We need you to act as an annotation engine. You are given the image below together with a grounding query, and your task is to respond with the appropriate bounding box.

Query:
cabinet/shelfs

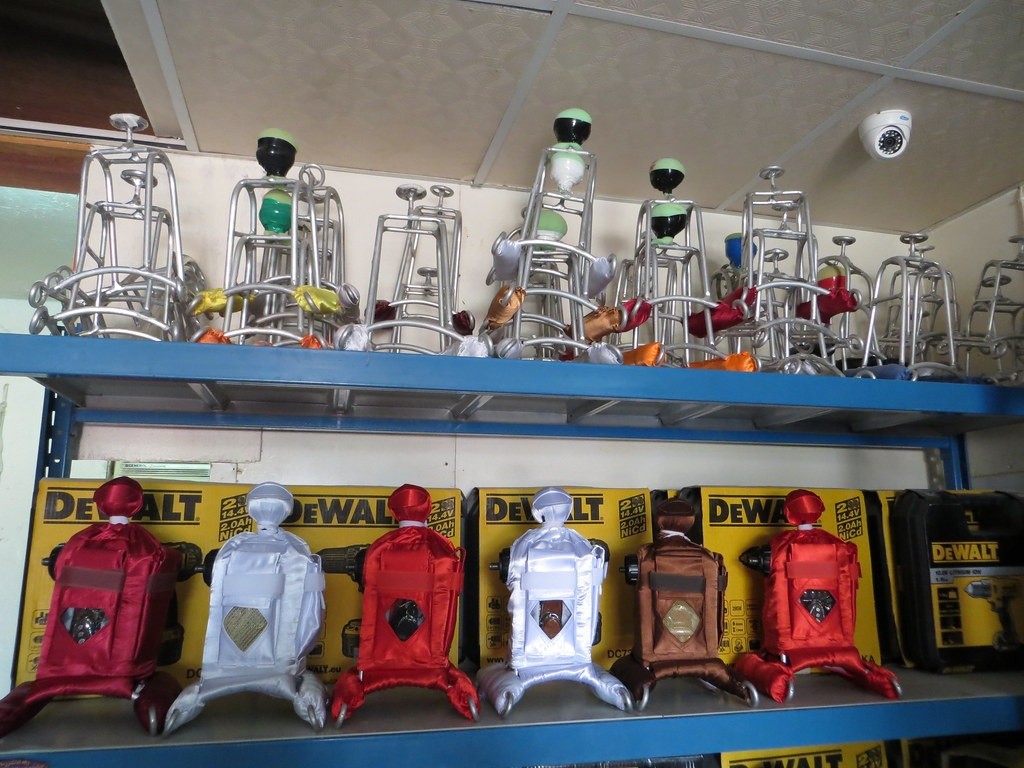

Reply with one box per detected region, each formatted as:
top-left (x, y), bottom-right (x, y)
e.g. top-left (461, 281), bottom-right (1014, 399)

top-left (0, 332), bottom-right (1024, 768)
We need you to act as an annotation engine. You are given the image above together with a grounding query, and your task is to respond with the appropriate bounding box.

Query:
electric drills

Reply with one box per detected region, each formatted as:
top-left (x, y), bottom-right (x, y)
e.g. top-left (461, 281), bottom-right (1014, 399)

top-left (41, 545), bottom-right (62, 581)
top-left (344, 549), bottom-right (364, 591)
top-left (489, 546), bottom-right (511, 583)
top-left (619, 554), bottom-right (639, 584)
top-left (737, 542), bottom-right (772, 575)
top-left (194, 547), bottom-right (220, 588)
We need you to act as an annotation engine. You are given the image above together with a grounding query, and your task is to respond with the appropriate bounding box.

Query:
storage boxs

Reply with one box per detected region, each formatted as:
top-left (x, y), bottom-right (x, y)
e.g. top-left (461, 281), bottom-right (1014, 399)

top-left (15, 477), bottom-right (258, 701)
top-left (466, 486), bottom-right (653, 671)
top-left (279, 486), bottom-right (461, 685)
top-left (679, 485), bottom-right (882, 674)
top-left (862, 490), bottom-right (1001, 667)
top-left (703, 740), bottom-right (888, 768)
top-left (650, 489), bottom-right (682, 542)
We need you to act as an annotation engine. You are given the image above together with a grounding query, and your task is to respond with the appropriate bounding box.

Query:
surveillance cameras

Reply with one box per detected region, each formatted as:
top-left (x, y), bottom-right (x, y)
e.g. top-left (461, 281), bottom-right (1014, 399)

top-left (858, 109), bottom-right (912, 160)
